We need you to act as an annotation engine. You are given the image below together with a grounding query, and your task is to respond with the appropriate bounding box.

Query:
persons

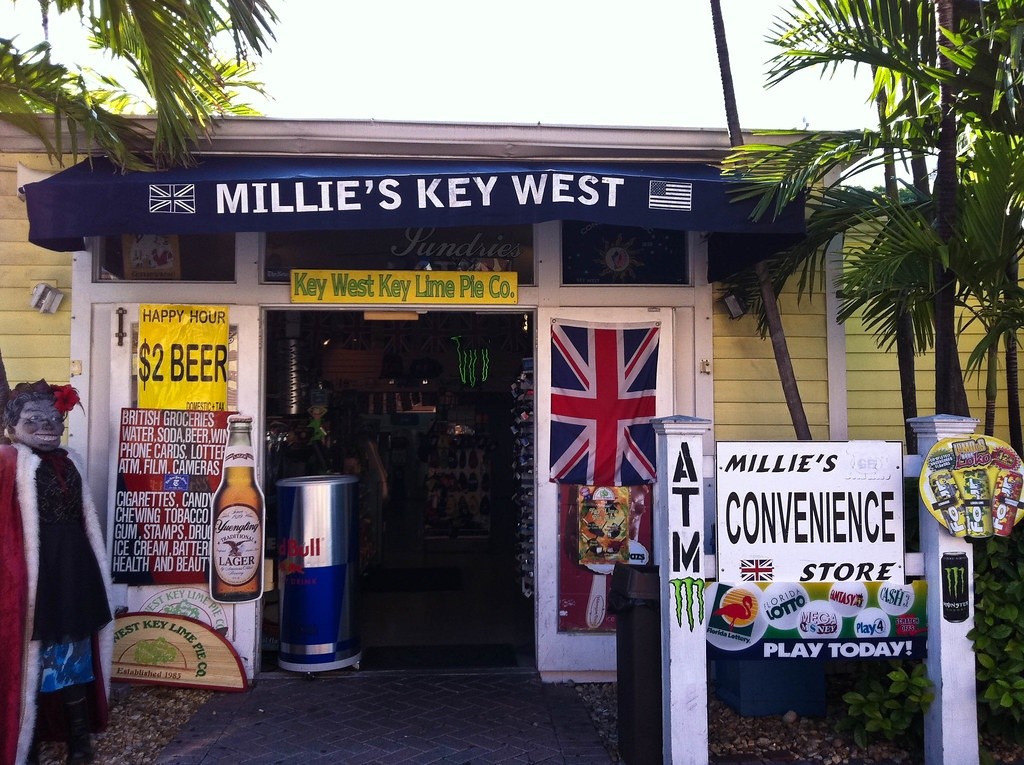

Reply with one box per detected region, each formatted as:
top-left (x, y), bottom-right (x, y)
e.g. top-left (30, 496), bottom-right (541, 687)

top-left (3, 383), bottom-right (65, 451)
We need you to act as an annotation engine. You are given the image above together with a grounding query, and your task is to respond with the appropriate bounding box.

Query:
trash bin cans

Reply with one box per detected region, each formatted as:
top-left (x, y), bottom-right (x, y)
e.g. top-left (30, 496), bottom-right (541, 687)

top-left (606, 558), bottom-right (663, 765)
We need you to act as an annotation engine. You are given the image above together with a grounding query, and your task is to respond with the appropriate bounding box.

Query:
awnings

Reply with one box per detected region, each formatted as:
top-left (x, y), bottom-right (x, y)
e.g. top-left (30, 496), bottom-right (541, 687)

top-left (18, 153), bottom-right (809, 254)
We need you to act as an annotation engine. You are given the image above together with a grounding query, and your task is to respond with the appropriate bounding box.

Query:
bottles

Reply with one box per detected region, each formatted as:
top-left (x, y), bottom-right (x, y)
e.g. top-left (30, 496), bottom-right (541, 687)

top-left (206, 416), bottom-right (266, 604)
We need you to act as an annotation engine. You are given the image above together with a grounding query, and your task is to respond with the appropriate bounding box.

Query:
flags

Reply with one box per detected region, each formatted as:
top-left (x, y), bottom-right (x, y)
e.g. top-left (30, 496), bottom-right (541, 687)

top-left (549, 316), bottom-right (660, 486)
